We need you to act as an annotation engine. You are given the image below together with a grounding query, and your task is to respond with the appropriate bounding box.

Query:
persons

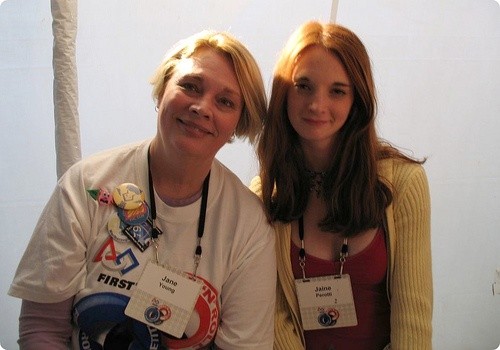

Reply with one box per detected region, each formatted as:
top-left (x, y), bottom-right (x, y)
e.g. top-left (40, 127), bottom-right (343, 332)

top-left (247, 19), bottom-right (434, 350)
top-left (6, 29), bottom-right (277, 350)
